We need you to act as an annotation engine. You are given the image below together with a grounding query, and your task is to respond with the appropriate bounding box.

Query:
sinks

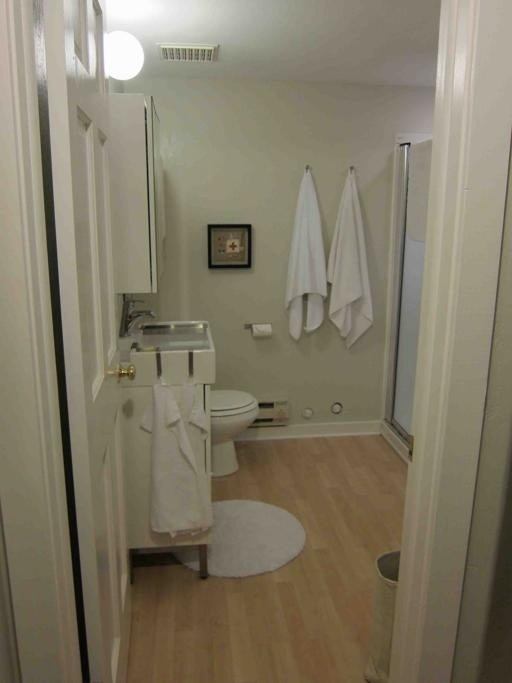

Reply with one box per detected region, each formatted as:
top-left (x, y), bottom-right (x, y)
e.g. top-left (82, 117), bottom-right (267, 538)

top-left (141, 323), bottom-right (206, 335)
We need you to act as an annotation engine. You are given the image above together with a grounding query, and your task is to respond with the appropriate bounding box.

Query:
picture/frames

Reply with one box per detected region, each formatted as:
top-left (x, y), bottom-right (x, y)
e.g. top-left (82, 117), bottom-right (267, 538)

top-left (208, 222), bottom-right (252, 271)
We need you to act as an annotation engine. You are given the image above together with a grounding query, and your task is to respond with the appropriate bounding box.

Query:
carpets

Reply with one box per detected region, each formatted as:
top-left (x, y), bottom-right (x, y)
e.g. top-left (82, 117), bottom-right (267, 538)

top-left (167, 497), bottom-right (307, 576)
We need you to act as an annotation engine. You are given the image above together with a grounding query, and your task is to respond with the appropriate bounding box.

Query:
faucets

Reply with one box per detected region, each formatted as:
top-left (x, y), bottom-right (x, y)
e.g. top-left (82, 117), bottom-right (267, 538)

top-left (121, 300), bottom-right (156, 337)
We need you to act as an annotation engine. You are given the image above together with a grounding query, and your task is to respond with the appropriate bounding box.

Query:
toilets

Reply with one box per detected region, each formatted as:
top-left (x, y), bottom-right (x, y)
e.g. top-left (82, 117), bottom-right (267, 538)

top-left (209, 389), bottom-right (259, 478)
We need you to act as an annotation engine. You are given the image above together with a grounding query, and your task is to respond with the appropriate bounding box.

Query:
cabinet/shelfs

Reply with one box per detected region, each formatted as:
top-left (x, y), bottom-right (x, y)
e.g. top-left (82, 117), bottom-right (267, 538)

top-left (111, 91), bottom-right (163, 293)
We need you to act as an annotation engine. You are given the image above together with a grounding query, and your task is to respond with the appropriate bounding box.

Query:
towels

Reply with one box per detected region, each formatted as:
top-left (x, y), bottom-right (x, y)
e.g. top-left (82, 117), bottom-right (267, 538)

top-left (282, 161), bottom-right (372, 351)
top-left (138, 370), bottom-right (211, 537)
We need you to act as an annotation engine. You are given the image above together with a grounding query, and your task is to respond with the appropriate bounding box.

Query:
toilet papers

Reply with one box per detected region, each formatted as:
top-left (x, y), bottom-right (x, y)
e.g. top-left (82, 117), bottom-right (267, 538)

top-left (251, 323), bottom-right (272, 337)
